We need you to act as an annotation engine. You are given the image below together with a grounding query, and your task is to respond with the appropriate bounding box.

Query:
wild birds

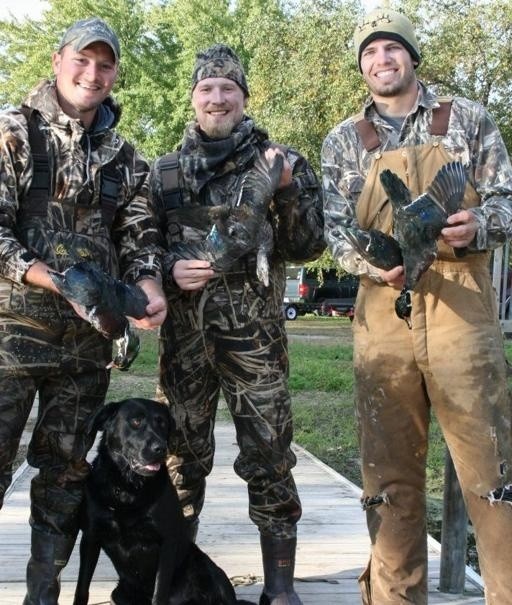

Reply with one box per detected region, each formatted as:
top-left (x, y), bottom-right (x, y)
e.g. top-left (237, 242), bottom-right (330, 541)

top-left (172, 150), bottom-right (285, 290)
top-left (334, 162), bottom-right (468, 331)
top-left (44, 230), bottom-right (150, 371)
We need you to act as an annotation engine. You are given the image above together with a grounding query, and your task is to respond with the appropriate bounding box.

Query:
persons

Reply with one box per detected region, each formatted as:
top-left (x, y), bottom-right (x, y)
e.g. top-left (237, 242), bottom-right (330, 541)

top-left (314, 7), bottom-right (511, 604)
top-left (0, 13), bottom-right (173, 605)
top-left (136, 40), bottom-right (328, 605)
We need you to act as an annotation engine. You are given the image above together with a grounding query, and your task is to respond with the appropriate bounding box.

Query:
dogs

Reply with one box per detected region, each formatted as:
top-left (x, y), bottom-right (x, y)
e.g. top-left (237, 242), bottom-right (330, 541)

top-left (72, 396), bottom-right (271, 604)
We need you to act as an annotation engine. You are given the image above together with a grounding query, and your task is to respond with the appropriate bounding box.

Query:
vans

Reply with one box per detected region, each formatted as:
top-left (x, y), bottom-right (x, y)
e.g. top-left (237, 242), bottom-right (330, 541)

top-left (282, 266), bottom-right (359, 307)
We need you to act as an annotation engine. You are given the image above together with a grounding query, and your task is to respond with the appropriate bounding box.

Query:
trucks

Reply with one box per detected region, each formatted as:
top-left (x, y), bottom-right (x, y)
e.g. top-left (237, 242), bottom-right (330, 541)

top-left (320, 298), bottom-right (355, 322)
top-left (280, 295), bottom-right (308, 321)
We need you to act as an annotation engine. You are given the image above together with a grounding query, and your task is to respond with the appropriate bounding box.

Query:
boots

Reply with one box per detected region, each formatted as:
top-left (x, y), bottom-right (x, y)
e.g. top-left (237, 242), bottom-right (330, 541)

top-left (258, 536), bottom-right (301, 605)
top-left (22, 525), bottom-right (77, 605)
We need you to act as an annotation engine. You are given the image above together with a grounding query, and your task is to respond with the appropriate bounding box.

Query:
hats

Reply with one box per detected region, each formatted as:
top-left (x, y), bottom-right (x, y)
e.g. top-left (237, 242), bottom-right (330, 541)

top-left (58, 18), bottom-right (121, 63)
top-left (354, 8), bottom-right (420, 74)
top-left (190, 43), bottom-right (250, 96)
